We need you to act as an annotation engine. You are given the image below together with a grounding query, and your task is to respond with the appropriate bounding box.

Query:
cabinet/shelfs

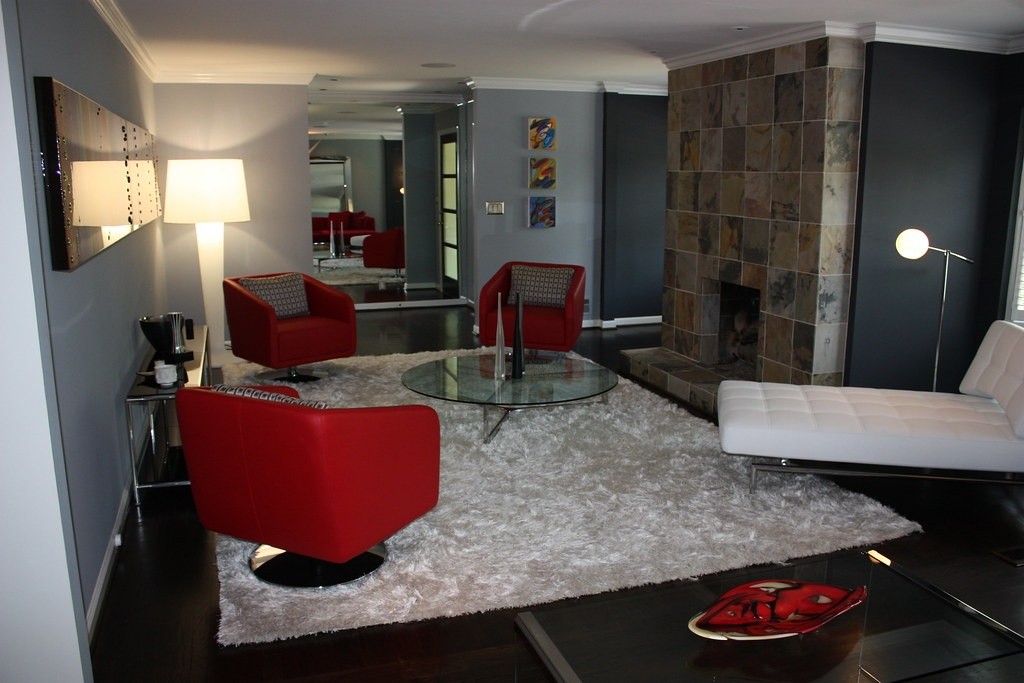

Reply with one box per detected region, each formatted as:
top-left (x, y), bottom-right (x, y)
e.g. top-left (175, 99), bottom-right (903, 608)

top-left (512, 546), bottom-right (1023, 683)
top-left (125, 324), bottom-right (210, 525)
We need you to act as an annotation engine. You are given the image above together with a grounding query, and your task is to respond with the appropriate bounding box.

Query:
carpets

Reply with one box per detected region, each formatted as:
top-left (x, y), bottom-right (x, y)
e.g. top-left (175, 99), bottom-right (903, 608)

top-left (217, 345), bottom-right (922, 643)
top-left (313, 249), bottom-right (405, 285)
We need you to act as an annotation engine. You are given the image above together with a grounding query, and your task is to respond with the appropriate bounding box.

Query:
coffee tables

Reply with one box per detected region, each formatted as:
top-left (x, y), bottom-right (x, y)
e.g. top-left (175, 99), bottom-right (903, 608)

top-left (312, 251), bottom-right (363, 271)
top-left (401, 353), bottom-right (618, 443)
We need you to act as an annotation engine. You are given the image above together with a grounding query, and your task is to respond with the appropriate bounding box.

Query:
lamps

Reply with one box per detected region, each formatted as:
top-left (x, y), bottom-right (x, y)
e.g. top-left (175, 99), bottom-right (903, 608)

top-left (69, 158), bottom-right (162, 248)
top-left (164, 157), bottom-right (252, 371)
top-left (895, 228), bottom-right (973, 392)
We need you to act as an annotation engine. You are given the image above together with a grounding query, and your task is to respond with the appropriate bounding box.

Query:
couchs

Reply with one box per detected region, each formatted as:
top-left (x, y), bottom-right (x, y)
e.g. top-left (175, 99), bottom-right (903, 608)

top-left (176, 381), bottom-right (440, 587)
top-left (312, 214), bottom-right (375, 245)
top-left (224, 270), bottom-right (357, 382)
top-left (715, 318), bottom-right (1023, 493)
top-left (363, 228), bottom-right (406, 278)
top-left (478, 260), bottom-right (588, 365)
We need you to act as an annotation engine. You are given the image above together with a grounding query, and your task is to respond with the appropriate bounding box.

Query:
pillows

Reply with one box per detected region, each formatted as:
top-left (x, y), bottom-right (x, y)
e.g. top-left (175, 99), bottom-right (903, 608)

top-left (239, 272), bottom-right (309, 318)
top-left (210, 383), bottom-right (331, 411)
top-left (327, 210), bottom-right (352, 230)
top-left (349, 211), bottom-right (365, 229)
top-left (509, 265), bottom-right (576, 308)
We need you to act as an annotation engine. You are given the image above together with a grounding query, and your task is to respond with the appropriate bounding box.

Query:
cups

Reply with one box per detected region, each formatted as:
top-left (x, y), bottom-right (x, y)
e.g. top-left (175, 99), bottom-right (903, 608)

top-left (154, 365), bottom-right (177, 386)
